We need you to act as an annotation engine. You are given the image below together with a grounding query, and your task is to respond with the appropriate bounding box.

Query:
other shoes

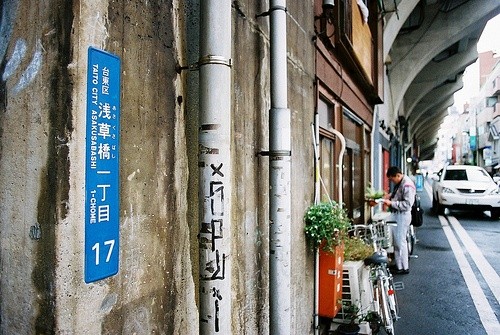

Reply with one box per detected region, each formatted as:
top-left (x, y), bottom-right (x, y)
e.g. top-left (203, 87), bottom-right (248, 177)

top-left (393, 269), bottom-right (408, 275)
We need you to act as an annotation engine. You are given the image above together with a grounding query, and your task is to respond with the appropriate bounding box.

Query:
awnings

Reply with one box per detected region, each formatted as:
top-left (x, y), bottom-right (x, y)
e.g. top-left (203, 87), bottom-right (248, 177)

top-left (494, 164), bottom-right (500, 169)
top-left (490, 162), bottom-right (498, 165)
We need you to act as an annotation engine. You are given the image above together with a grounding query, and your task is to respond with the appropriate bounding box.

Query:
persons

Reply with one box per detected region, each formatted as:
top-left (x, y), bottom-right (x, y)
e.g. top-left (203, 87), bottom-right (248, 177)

top-left (380, 166), bottom-right (416, 274)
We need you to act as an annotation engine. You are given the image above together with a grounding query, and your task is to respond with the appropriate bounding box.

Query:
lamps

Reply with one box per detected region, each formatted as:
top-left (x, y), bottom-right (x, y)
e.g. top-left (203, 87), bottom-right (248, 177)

top-left (314, 0), bottom-right (335, 24)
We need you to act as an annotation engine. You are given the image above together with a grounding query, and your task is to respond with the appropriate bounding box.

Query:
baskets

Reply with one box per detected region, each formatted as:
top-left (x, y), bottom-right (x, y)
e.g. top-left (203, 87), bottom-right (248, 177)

top-left (356, 224), bottom-right (389, 249)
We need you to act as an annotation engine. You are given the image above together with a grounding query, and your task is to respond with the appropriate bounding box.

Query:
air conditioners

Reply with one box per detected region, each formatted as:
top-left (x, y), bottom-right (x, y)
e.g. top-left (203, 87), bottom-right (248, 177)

top-left (353, 224), bottom-right (377, 255)
top-left (332, 260), bottom-right (374, 324)
top-left (373, 219), bottom-right (393, 248)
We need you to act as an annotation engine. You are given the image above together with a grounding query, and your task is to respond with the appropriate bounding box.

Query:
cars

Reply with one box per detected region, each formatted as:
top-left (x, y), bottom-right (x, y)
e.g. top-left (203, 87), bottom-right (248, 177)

top-left (428, 164), bottom-right (500, 221)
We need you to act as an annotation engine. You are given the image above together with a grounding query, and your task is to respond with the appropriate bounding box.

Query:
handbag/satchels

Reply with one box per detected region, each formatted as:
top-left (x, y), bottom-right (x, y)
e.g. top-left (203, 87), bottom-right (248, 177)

top-left (412, 206), bottom-right (424, 226)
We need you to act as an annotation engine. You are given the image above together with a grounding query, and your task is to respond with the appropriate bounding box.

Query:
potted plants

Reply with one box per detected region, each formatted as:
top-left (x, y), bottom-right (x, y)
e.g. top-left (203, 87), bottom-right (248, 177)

top-left (365, 183), bottom-right (385, 206)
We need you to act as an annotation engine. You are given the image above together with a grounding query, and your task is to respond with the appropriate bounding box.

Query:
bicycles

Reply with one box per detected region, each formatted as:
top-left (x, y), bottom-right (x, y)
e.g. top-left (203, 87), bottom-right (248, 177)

top-left (347, 219), bottom-right (404, 335)
top-left (406, 223), bottom-right (420, 260)
